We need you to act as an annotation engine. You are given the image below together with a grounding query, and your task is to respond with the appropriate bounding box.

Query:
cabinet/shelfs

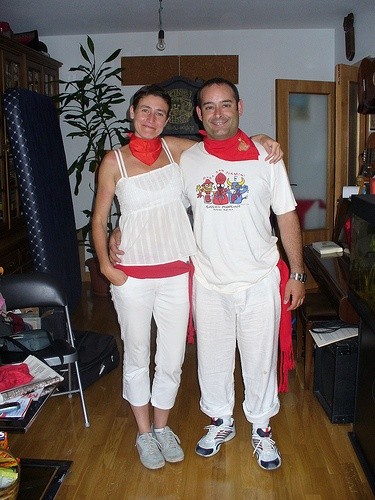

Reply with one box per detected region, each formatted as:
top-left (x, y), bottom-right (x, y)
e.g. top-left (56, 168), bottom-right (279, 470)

top-left (0, 34), bottom-right (63, 278)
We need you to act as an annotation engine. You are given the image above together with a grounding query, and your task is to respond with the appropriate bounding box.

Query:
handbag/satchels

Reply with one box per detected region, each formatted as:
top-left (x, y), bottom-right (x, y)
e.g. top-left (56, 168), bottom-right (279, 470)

top-left (57, 330), bottom-right (120, 395)
top-left (0, 322), bottom-right (64, 372)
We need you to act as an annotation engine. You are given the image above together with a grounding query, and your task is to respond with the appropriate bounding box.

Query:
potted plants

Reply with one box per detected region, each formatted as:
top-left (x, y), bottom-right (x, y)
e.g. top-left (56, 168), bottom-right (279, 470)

top-left (46, 35), bottom-right (135, 297)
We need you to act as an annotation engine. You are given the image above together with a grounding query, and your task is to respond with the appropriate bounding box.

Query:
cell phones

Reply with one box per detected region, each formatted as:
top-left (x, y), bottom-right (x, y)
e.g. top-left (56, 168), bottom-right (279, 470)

top-left (0, 402), bottom-right (20, 414)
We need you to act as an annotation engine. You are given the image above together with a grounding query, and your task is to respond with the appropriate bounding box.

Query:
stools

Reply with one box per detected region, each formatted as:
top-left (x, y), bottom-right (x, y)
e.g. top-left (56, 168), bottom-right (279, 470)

top-left (296, 292), bottom-right (338, 390)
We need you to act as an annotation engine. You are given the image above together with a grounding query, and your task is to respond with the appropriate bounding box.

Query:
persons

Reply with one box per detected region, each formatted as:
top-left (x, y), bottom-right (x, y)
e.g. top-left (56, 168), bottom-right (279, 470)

top-left (92, 86), bottom-right (286, 471)
top-left (107, 79), bottom-right (306, 473)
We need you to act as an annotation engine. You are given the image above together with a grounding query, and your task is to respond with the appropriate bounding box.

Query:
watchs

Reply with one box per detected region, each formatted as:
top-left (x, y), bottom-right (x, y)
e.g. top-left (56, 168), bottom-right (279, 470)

top-left (289, 273), bottom-right (308, 283)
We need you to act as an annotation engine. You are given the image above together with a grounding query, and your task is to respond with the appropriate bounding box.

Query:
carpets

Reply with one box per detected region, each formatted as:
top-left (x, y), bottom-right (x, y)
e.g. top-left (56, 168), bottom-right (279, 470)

top-left (16, 458), bottom-right (73, 500)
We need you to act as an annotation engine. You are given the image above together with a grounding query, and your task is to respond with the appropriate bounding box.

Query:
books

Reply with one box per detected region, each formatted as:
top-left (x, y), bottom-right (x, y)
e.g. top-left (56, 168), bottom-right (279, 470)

top-left (311, 240), bottom-right (343, 254)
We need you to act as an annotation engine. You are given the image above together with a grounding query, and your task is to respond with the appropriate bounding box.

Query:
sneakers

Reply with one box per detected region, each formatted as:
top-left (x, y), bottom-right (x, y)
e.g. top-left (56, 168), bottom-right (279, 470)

top-left (251, 424), bottom-right (282, 470)
top-left (151, 423), bottom-right (185, 462)
top-left (194, 417), bottom-right (236, 458)
top-left (133, 430), bottom-right (170, 470)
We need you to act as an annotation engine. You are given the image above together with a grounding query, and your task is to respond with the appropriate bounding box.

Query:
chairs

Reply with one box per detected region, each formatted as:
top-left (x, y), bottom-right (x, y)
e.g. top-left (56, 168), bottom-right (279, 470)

top-left (0, 272), bottom-right (91, 429)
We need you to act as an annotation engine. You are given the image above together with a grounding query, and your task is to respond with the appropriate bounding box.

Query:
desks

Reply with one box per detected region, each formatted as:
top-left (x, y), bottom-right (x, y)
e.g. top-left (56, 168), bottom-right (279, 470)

top-left (303, 243), bottom-right (360, 325)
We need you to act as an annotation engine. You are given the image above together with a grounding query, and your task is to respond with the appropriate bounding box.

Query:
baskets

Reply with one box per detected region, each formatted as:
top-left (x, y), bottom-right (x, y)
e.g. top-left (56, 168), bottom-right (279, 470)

top-left (0, 448), bottom-right (21, 500)
